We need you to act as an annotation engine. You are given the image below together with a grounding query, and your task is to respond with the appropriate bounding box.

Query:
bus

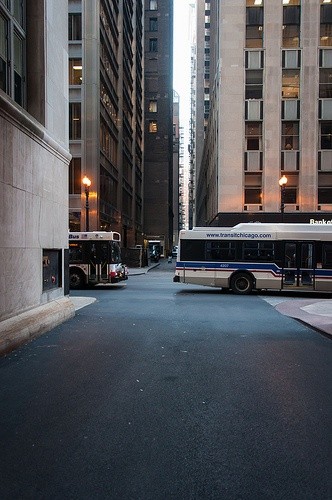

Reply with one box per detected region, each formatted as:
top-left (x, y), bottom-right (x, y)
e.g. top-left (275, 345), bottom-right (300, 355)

top-left (68, 231), bottom-right (129, 290)
top-left (172, 222), bottom-right (332, 296)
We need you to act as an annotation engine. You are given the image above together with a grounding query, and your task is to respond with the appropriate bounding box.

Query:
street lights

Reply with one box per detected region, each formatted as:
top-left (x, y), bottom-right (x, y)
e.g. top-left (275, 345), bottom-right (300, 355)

top-left (81, 176), bottom-right (92, 231)
top-left (278, 175), bottom-right (288, 222)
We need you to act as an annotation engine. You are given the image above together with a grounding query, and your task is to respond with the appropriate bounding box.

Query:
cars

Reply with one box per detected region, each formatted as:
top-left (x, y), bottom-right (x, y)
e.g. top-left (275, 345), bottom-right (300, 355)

top-left (172, 245), bottom-right (178, 258)
top-left (150, 250), bottom-right (160, 263)
